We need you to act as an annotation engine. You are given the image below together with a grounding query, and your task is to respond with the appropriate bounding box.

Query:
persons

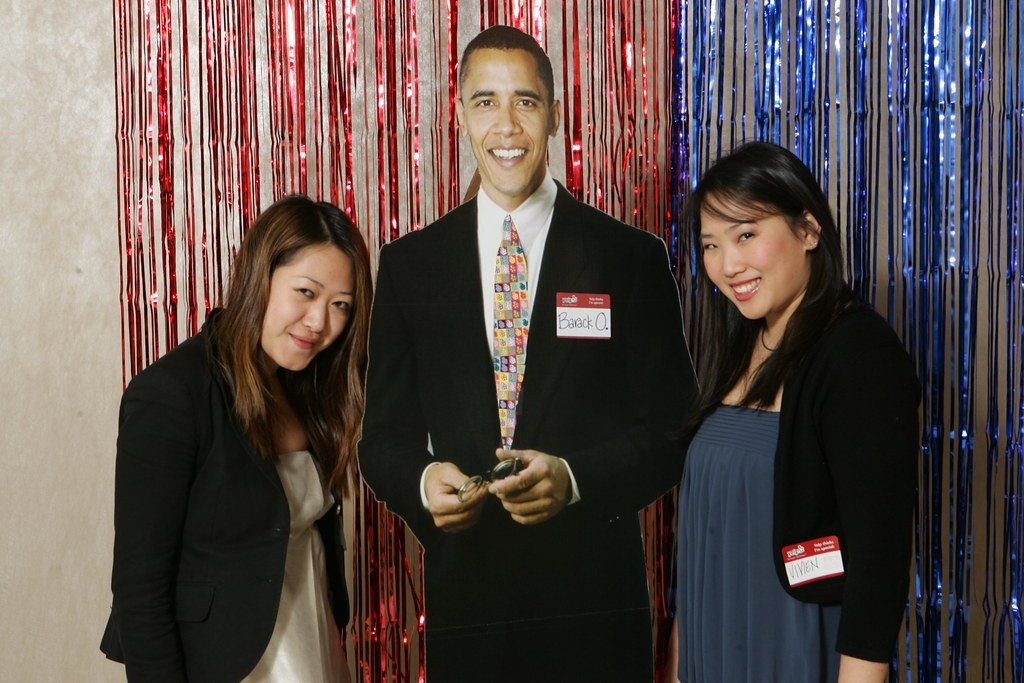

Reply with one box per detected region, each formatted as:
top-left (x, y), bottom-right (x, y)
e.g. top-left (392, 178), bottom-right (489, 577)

top-left (97, 193), bottom-right (372, 683)
top-left (360, 25), bottom-right (710, 683)
top-left (662, 139), bottom-right (924, 683)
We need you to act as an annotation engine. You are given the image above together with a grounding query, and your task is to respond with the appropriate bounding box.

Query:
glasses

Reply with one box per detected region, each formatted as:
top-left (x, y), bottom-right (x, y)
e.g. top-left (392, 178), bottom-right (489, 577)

top-left (457, 458), bottom-right (520, 502)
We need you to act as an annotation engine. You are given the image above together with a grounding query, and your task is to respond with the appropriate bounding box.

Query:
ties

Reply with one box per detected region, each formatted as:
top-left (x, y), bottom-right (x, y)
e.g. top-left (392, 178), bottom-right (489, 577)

top-left (493, 214), bottom-right (531, 451)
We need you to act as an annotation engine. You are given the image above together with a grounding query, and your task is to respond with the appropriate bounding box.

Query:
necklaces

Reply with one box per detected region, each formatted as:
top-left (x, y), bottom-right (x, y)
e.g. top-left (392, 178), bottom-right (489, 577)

top-left (761, 322), bottom-right (777, 352)
